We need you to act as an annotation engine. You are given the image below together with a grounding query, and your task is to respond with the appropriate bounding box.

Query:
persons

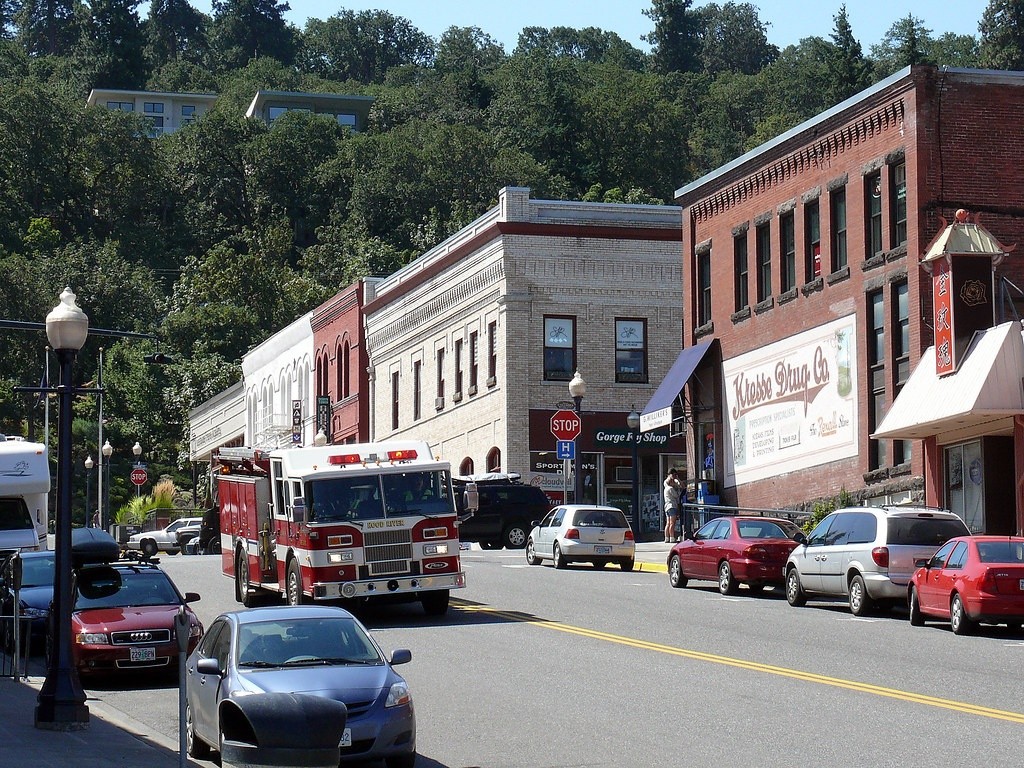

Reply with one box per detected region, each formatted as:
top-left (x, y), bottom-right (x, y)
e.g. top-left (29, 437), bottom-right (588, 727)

top-left (664, 467), bottom-right (684, 543)
top-left (93, 510), bottom-right (99, 528)
top-left (401, 475), bottom-right (433, 501)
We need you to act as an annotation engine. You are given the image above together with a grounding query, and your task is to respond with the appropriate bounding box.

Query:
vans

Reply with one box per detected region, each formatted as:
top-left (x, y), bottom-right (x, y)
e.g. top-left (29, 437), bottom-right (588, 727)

top-left (0, 431), bottom-right (51, 565)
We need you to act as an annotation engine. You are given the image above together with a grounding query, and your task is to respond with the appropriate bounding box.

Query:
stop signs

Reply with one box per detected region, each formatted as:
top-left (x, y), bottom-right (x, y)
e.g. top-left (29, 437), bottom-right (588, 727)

top-left (131, 468), bottom-right (148, 485)
top-left (550, 410), bottom-right (581, 442)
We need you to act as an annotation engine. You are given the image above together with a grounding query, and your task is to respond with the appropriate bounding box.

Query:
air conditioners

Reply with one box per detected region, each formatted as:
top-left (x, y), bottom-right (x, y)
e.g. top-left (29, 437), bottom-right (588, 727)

top-left (435, 396), bottom-right (444, 409)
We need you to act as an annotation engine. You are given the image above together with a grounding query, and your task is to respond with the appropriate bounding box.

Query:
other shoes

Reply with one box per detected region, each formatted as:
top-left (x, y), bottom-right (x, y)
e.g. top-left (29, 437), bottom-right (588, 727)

top-left (670, 540), bottom-right (680, 543)
top-left (665, 540), bottom-right (670, 543)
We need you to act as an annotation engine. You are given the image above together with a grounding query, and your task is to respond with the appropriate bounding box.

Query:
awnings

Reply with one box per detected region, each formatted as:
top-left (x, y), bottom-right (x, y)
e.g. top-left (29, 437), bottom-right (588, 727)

top-left (640, 337), bottom-right (714, 432)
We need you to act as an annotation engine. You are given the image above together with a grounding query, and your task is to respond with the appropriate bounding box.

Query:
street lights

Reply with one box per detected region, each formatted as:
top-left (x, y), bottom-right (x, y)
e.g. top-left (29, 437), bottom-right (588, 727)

top-left (33, 282), bottom-right (92, 731)
top-left (101, 438), bottom-right (113, 533)
top-left (568, 371), bottom-right (588, 505)
top-left (84, 454), bottom-right (94, 528)
top-left (132, 441), bottom-right (143, 498)
top-left (626, 403), bottom-right (645, 543)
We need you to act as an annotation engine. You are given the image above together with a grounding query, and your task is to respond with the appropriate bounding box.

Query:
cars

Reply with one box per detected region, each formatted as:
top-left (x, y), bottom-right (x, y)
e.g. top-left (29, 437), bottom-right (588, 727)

top-left (44, 527), bottom-right (204, 683)
top-left (905, 536), bottom-right (1024, 636)
top-left (0, 550), bottom-right (57, 657)
top-left (525, 504), bottom-right (636, 573)
top-left (184, 604), bottom-right (416, 768)
top-left (666, 515), bottom-right (808, 597)
top-left (127, 517), bottom-right (203, 557)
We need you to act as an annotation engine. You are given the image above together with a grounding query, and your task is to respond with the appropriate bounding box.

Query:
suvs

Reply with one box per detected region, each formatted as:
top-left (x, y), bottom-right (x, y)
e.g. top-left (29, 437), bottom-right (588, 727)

top-left (785, 504), bottom-right (973, 618)
top-left (441, 479), bottom-right (553, 550)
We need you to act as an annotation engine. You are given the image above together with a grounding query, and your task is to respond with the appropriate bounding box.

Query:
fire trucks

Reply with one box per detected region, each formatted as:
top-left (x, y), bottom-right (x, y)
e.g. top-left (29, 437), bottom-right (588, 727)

top-left (212, 437), bottom-right (467, 618)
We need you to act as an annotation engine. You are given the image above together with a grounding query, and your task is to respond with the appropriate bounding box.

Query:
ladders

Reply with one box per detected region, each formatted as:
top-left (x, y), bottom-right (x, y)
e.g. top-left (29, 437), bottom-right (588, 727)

top-left (219, 445), bottom-right (279, 458)
